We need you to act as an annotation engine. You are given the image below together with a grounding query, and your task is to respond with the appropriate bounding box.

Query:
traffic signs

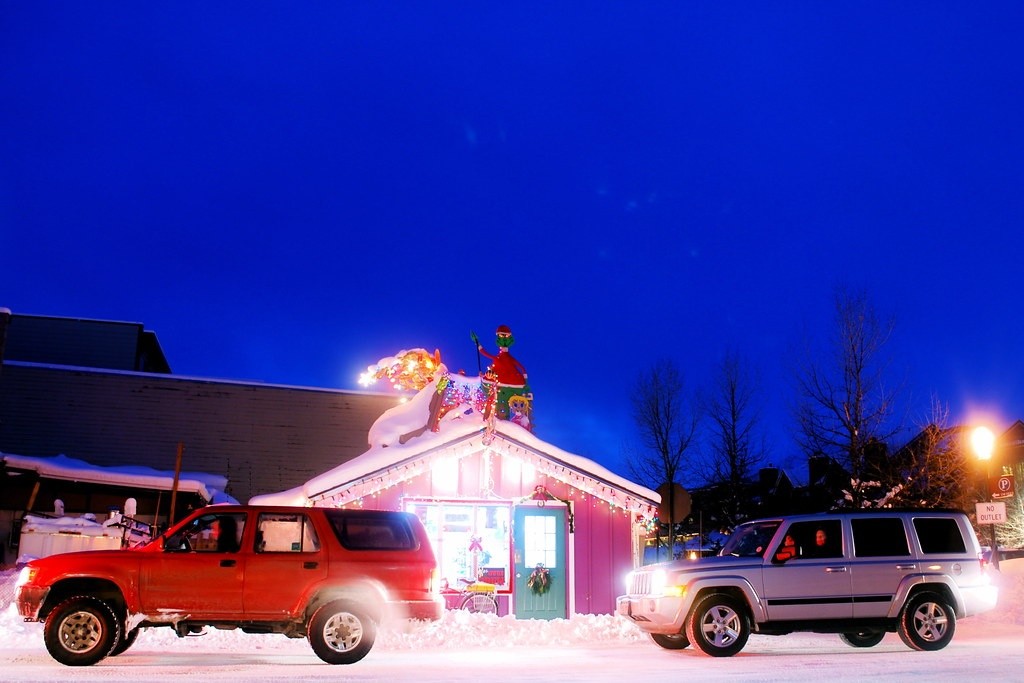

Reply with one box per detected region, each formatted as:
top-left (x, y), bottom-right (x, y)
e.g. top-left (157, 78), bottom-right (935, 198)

top-left (992, 474), bottom-right (1015, 499)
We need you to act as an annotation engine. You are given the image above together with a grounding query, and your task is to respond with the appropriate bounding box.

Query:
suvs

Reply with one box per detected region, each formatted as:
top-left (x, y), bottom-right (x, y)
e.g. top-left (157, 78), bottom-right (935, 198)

top-left (17, 504), bottom-right (445, 668)
top-left (616, 508), bottom-right (1005, 659)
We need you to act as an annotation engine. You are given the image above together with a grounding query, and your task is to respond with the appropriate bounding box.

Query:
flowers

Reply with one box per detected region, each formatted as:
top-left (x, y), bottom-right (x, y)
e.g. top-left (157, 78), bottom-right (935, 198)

top-left (526, 564), bottom-right (555, 596)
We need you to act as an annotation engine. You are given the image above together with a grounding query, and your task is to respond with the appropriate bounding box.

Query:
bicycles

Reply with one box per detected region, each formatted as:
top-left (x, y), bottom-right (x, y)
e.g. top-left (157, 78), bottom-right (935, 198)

top-left (441, 578), bottom-right (499, 616)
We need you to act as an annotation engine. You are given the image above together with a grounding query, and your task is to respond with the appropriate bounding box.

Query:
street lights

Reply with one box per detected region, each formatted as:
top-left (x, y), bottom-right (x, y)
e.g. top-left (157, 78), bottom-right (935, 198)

top-left (971, 426), bottom-right (1000, 571)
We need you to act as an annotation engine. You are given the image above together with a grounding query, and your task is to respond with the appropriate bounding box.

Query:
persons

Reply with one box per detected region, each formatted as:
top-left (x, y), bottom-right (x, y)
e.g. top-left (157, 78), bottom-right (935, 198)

top-left (815, 529), bottom-right (826, 546)
top-left (508, 393), bottom-right (531, 432)
top-left (210, 517), bottom-right (240, 553)
